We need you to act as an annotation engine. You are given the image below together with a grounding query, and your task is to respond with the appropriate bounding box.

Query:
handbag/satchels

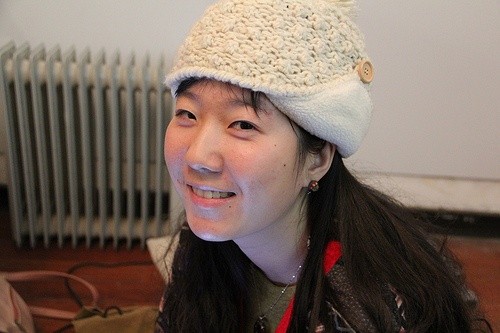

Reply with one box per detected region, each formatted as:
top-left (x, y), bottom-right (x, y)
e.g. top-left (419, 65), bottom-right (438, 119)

top-left (0, 273), bottom-right (36, 333)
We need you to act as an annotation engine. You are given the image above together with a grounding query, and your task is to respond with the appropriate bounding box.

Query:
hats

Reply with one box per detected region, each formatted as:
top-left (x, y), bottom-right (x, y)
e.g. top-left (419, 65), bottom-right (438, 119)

top-left (163, 1), bottom-right (374, 158)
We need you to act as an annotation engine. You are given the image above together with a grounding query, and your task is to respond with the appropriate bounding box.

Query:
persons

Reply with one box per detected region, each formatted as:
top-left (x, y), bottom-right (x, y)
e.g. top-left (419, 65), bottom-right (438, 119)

top-left (158, 1), bottom-right (494, 333)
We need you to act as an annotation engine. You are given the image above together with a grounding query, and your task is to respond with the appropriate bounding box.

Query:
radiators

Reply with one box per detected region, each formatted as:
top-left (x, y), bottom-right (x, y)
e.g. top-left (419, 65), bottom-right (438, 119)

top-left (0, 39), bottom-right (187, 252)
